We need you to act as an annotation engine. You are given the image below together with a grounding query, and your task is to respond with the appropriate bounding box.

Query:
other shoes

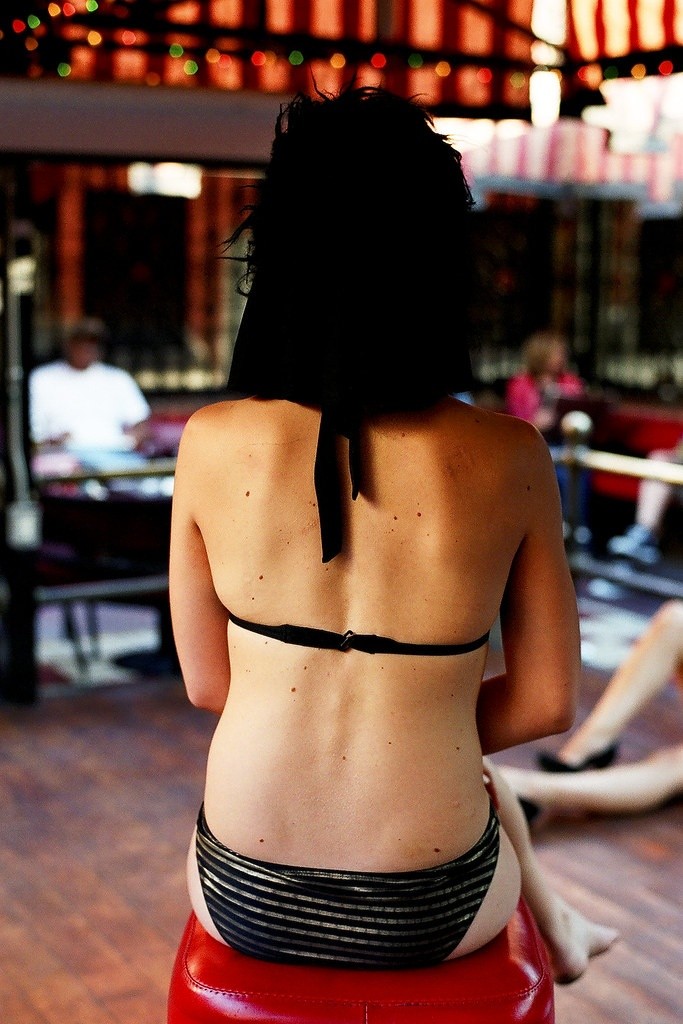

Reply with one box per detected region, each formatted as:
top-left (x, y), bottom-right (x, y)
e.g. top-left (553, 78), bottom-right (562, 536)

top-left (539, 745), bottom-right (613, 773)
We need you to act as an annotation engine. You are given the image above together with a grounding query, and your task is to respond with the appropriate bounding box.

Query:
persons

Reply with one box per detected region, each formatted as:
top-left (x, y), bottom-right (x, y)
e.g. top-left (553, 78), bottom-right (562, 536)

top-left (585, 442), bottom-right (683, 601)
top-left (28, 320), bottom-right (154, 677)
top-left (495, 596), bottom-right (683, 827)
top-left (510, 329), bottom-right (591, 540)
top-left (168, 86), bottom-right (622, 989)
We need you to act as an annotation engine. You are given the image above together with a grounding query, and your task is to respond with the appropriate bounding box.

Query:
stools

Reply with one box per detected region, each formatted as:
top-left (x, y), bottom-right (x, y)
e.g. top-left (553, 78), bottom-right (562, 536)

top-left (166, 895), bottom-right (557, 1023)
